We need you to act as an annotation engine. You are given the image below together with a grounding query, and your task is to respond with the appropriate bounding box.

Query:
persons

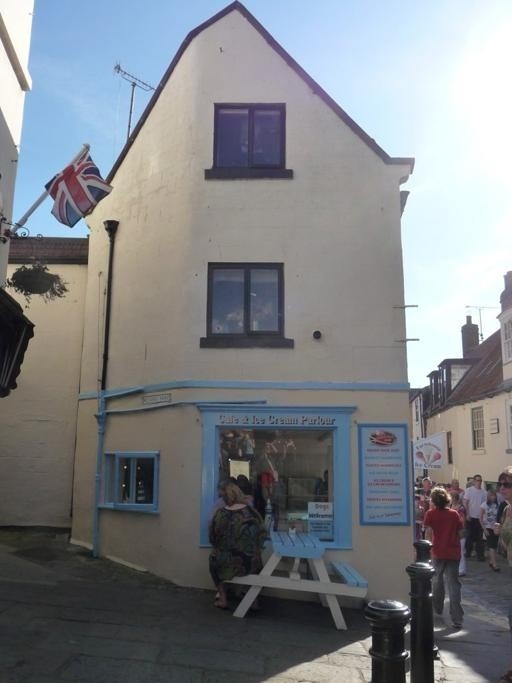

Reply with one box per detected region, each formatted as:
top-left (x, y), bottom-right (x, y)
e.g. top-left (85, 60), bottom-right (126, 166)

top-left (414, 465), bottom-right (512, 683)
top-left (315, 470), bottom-right (328, 502)
top-left (208, 475), bottom-right (267, 610)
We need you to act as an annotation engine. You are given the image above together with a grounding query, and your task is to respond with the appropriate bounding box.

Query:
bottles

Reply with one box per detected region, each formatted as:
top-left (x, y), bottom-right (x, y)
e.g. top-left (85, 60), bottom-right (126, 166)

top-left (264, 498), bottom-right (274, 538)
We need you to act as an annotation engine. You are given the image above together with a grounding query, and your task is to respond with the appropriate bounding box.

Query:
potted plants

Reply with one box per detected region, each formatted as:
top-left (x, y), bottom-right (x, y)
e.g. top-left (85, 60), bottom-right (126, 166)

top-left (3, 263), bottom-right (70, 309)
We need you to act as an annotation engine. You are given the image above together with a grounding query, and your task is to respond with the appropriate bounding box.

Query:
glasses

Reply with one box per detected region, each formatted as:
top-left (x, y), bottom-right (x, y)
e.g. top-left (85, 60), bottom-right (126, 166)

top-left (496, 481), bottom-right (512, 488)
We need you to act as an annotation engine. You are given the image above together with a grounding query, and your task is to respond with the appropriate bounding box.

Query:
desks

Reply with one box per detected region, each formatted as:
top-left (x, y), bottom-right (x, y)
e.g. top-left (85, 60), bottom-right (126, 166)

top-left (233, 531), bottom-right (348, 630)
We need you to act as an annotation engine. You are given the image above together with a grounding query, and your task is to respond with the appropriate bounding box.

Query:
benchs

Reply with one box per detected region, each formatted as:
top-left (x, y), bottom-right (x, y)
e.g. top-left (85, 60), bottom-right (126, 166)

top-left (327, 561), bottom-right (368, 599)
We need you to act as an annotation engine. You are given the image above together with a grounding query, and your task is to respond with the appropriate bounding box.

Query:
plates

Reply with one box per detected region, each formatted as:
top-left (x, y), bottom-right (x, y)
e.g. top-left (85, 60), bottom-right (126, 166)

top-left (368, 431), bottom-right (397, 447)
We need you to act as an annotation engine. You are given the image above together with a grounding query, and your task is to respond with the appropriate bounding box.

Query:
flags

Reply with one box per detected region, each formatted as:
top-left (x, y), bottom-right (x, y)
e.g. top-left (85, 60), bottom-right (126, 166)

top-left (44, 148), bottom-right (114, 228)
